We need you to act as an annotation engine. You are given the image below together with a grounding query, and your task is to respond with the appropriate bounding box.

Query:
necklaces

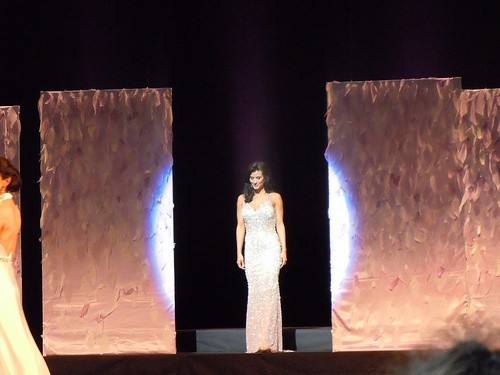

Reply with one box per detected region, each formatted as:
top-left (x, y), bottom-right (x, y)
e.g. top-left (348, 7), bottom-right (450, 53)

top-left (0, 193), bottom-right (13, 201)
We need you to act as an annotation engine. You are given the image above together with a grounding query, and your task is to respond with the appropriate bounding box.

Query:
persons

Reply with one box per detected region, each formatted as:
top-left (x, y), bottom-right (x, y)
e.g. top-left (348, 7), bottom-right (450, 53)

top-left (236, 162), bottom-right (286, 353)
top-left (0, 157), bottom-right (51, 375)
